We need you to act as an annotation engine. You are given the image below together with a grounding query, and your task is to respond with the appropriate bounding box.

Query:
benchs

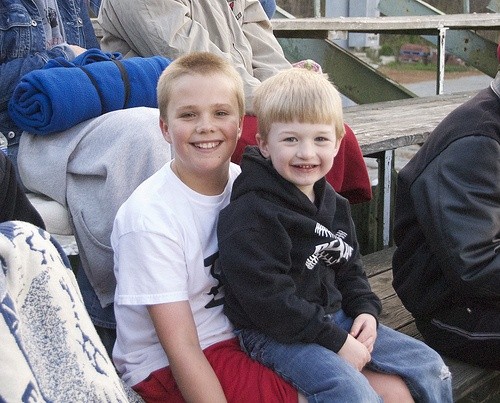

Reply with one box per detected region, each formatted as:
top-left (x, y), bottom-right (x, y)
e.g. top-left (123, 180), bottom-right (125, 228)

top-left (50, 13), bottom-right (500, 403)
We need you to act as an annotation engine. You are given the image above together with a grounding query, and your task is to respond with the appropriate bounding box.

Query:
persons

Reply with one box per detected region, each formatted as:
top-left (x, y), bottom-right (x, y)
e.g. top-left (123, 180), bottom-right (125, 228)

top-left (0, 150), bottom-right (47, 231)
top-left (393, 41), bottom-right (500, 371)
top-left (97, 0), bottom-right (372, 205)
top-left (112, 53), bottom-right (414, 403)
top-left (0, 0), bottom-right (172, 359)
top-left (217, 66), bottom-right (453, 403)
